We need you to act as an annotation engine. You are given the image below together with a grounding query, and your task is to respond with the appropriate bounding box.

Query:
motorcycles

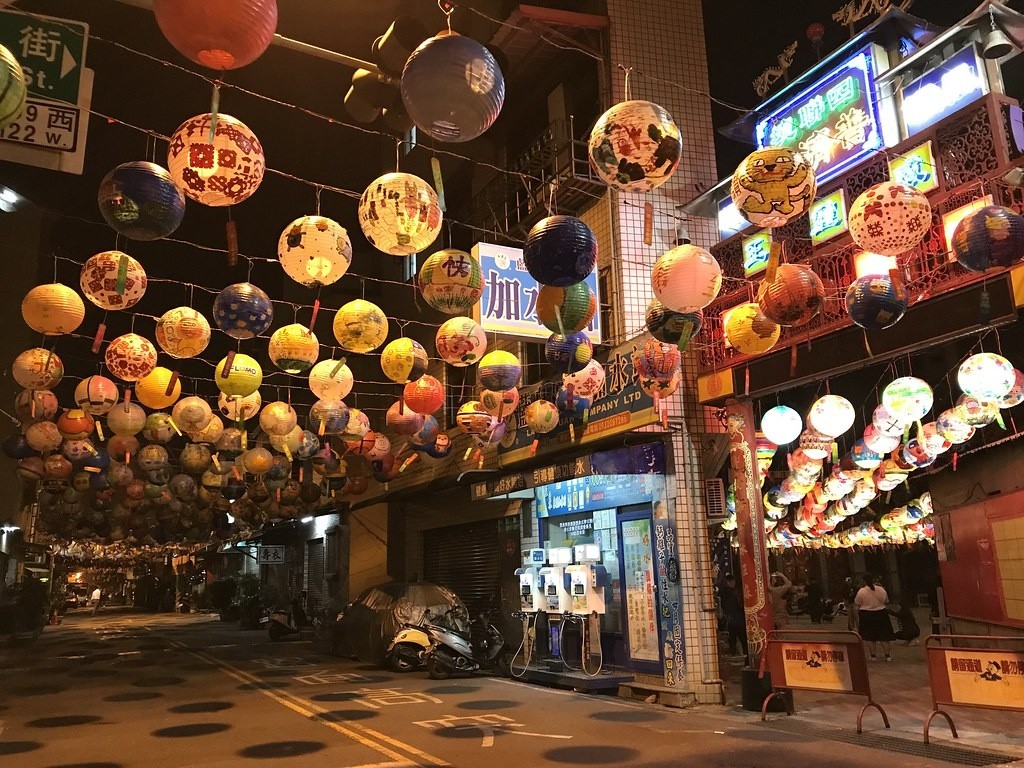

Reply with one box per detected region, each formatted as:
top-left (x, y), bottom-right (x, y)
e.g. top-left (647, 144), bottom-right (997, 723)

top-left (382, 591), bottom-right (514, 679)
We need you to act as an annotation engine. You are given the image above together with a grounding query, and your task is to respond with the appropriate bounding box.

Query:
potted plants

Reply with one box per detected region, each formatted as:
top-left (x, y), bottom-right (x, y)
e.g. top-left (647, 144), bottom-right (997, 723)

top-left (241, 574), bottom-right (263, 628)
top-left (208, 576), bottom-right (241, 622)
top-left (0, 572), bottom-right (51, 642)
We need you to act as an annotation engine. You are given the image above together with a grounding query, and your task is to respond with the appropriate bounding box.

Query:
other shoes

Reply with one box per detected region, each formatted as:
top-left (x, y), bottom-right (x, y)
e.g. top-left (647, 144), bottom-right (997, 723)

top-left (868, 656), bottom-right (876, 662)
top-left (887, 657), bottom-right (891, 662)
top-left (92, 615), bottom-right (94, 617)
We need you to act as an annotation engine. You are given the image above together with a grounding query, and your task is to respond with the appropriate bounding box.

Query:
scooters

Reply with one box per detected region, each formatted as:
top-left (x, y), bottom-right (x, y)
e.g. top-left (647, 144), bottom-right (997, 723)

top-left (259, 589), bottom-right (321, 644)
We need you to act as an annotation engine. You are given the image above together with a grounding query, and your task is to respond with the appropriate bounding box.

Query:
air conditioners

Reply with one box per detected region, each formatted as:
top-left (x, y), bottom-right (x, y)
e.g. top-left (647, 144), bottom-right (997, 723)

top-left (704, 477), bottom-right (728, 518)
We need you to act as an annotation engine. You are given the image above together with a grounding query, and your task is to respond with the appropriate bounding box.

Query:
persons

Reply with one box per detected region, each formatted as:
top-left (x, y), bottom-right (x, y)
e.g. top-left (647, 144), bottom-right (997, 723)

top-left (712, 575), bottom-right (751, 666)
top-left (763, 570), bottom-right (795, 638)
top-left (90, 587), bottom-right (101, 617)
top-left (887, 599), bottom-right (920, 646)
top-left (855, 572), bottom-right (897, 663)
top-left (797, 585), bottom-right (824, 625)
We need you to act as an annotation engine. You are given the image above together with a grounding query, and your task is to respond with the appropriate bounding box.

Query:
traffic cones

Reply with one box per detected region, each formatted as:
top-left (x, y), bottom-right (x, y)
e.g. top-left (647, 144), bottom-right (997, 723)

top-left (51, 609), bottom-right (58, 625)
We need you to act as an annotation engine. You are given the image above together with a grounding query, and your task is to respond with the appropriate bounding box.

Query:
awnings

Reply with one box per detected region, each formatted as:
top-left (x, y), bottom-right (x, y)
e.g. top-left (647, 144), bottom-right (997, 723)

top-left (471, 441), bottom-right (664, 503)
top-left (217, 540), bottom-right (262, 553)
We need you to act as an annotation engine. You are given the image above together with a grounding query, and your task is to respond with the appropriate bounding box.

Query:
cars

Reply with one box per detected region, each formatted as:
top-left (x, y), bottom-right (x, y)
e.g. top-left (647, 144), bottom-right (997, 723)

top-left (327, 577), bottom-right (472, 667)
